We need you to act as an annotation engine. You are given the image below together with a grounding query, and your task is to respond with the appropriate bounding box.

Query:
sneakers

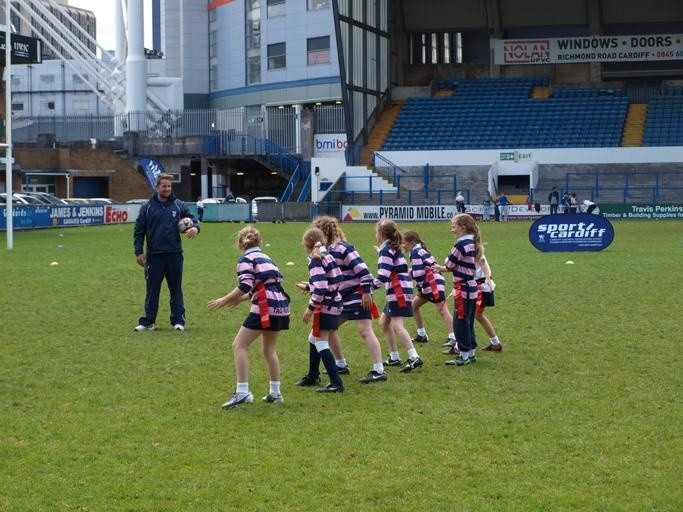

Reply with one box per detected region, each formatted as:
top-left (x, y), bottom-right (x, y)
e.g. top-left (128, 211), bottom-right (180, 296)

top-left (444, 356), bottom-right (471, 366)
top-left (316, 382), bottom-right (345, 393)
top-left (323, 364), bottom-right (350, 375)
top-left (296, 374), bottom-right (321, 385)
top-left (400, 358), bottom-right (423, 373)
top-left (383, 357), bottom-right (402, 366)
top-left (442, 337), bottom-right (456, 346)
top-left (262, 392), bottom-right (283, 403)
top-left (411, 333), bottom-right (429, 343)
top-left (221, 391), bottom-right (254, 409)
top-left (482, 343), bottom-right (502, 351)
top-left (442, 345), bottom-right (458, 355)
top-left (360, 371), bottom-right (388, 384)
top-left (135, 324), bottom-right (154, 331)
top-left (174, 324), bottom-right (184, 331)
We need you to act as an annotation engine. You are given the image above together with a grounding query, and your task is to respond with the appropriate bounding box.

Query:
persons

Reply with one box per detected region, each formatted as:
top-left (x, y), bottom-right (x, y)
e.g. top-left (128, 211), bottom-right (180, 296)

top-left (223, 191), bottom-right (236, 224)
top-left (133, 173), bottom-right (202, 332)
top-left (442, 213), bottom-right (482, 366)
top-left (296, 216), bottom-right (388, 385)
top-left (371, 219), bottom-right (422, 373)
top-left (206, 225), bottom-right (290, 408)
top-left (430, 243), bottom-right (502, 355)
top-left (454, 185), bottom-right (597, 222)
top-left (196, 196), bottom-right (205, 224)
top-left (401, 230), bottom-right (456, 347)
top-left (295, 227), bottom-right (344, 393)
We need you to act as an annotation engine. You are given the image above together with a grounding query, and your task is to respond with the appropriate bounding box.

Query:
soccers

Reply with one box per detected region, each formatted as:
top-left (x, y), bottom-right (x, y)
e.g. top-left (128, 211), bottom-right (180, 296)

top-left (178, 217), bottom-right (193, 233)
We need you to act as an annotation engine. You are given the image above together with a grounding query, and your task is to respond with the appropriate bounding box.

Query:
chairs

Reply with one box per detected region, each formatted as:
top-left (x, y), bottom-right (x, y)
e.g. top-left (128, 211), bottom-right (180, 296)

top-left (638, 78), bottom-right (683, 147)
top-left (370, 74), bottom-right (632, 168)
